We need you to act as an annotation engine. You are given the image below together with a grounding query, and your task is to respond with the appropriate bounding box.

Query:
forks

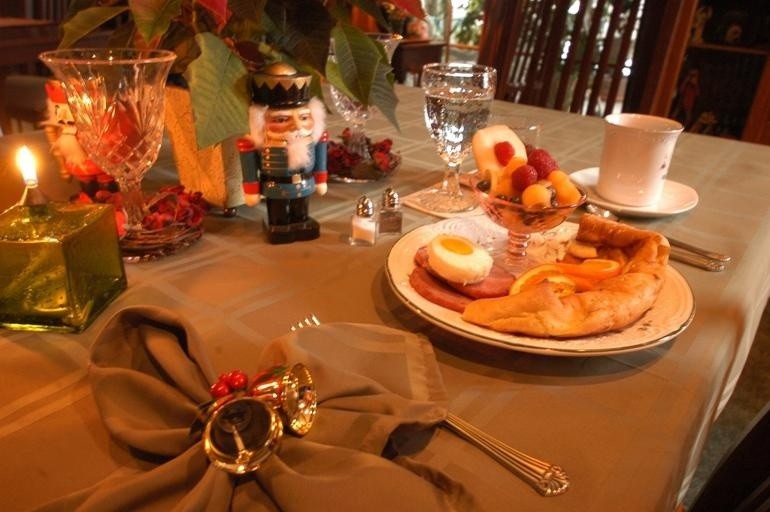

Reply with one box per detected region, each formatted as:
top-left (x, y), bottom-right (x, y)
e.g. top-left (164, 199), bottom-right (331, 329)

top-left (289, 312), bottom-right (572, 496)
top-left (587, 204), bottom-right (732, 262)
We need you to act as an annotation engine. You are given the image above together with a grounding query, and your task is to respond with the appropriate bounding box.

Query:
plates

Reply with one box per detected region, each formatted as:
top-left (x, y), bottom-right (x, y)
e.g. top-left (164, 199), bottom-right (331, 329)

top-left (383, 217), bottom-right (697, 357)
top-left (569, 166), bottom-right (700, 220)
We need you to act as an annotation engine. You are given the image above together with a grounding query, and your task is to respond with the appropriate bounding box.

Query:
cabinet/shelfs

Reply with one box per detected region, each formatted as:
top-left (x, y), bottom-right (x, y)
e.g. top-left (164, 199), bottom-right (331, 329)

top-left (633, 1), bottom-right (768, 146)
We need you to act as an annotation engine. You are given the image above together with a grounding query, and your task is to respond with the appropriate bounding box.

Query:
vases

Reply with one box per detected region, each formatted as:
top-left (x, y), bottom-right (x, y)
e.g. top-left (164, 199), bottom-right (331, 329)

top-left (162, 83), bottom-right (263, 209)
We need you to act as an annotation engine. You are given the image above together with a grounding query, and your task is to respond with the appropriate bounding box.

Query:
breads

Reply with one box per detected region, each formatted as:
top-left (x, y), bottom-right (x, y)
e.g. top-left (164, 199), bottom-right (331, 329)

top-left (462, 213), bottom-right (670, 337)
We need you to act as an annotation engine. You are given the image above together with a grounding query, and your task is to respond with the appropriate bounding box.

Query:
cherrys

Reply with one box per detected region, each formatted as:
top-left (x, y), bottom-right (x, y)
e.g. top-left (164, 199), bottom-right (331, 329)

top-left (210, 369), bottom-right (247, 400)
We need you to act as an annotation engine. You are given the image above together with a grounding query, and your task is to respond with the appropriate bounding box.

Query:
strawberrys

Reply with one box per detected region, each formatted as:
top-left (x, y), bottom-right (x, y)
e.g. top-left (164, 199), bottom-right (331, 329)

top-left (495, 141), bottom-right (559, 192)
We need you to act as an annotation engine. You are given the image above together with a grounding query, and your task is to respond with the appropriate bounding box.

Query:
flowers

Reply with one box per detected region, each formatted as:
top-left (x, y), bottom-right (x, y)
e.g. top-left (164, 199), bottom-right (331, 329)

top-left (52, 1), bottom-right (427, 152)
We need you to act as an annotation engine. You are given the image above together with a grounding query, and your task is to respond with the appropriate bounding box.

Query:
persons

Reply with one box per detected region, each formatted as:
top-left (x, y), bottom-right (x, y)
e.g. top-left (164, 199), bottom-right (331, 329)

top-left (232, 58), bottom-right (331, 250)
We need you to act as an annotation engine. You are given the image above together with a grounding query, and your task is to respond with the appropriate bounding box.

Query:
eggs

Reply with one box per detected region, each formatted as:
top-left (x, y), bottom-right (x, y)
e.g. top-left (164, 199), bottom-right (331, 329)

top-left (428, 235), bottom-right (492, 284)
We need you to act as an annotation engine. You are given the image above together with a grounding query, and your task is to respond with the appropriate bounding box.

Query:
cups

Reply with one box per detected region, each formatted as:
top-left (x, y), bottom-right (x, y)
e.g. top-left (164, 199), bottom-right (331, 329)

top-left (595, 113), bottom-right (685, 206)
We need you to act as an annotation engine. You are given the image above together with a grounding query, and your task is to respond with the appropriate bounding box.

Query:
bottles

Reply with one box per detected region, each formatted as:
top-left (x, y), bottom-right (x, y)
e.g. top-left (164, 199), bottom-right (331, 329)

top-left (347, 188), bottom-right (402, 247)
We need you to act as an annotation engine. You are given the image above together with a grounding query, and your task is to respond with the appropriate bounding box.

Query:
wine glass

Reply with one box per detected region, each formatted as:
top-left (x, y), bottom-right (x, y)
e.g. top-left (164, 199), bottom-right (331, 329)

top-left (416, 63), bottom-right (496, 213)
top-left (471, 173), bottom-right (589, 273)
top-left (326, 32), bottom-right (403, 183)
top-left (36, 48), bottom-right (179, 264)
